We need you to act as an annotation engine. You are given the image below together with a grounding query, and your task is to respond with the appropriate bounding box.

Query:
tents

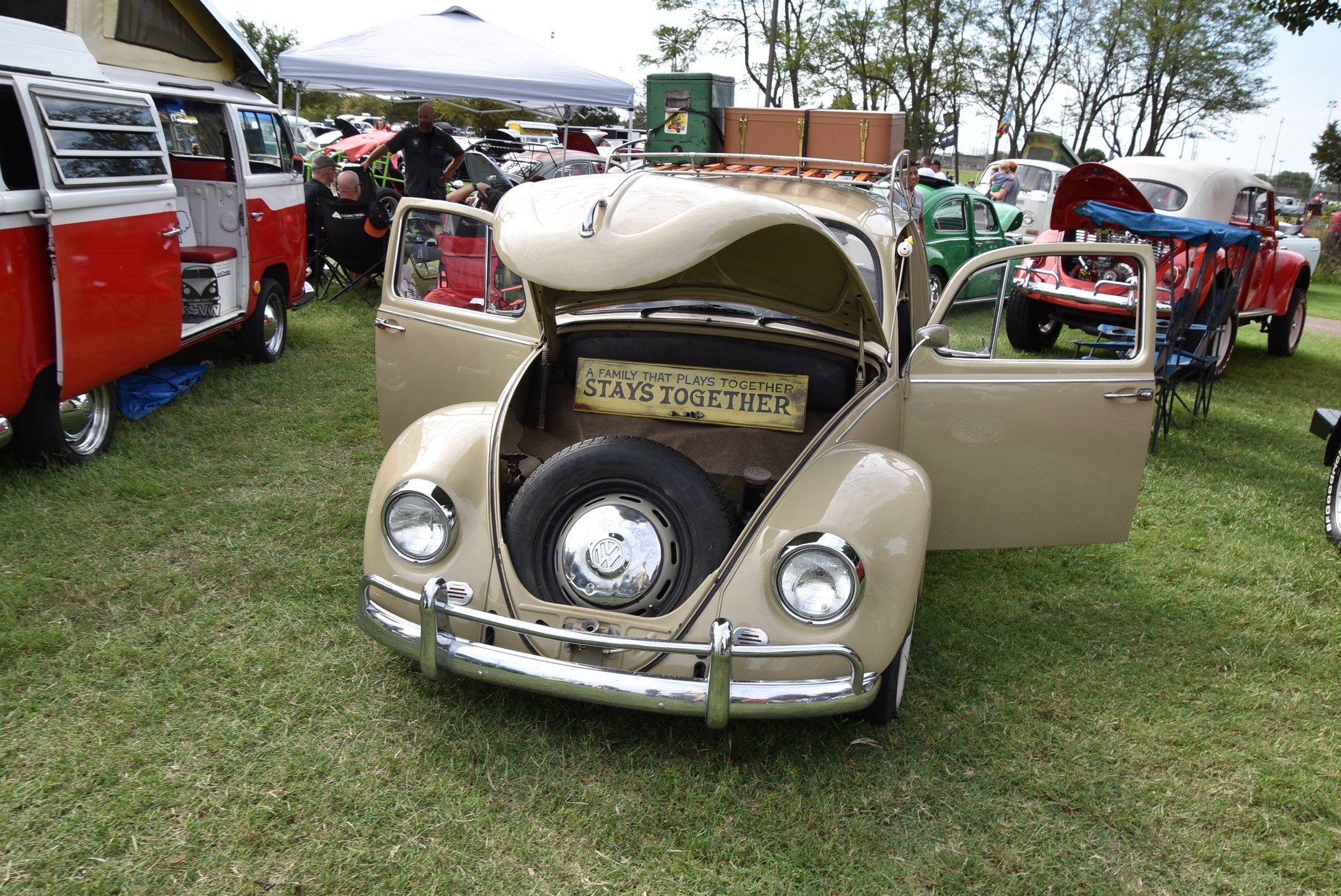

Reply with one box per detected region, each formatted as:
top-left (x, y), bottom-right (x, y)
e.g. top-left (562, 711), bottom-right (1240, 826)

top-left (275, 5), bottom-right (637, 173)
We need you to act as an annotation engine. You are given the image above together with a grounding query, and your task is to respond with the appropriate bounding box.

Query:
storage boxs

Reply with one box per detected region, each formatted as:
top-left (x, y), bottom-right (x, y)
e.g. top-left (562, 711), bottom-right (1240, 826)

top-left (644, 73), bottom-right (735, 165)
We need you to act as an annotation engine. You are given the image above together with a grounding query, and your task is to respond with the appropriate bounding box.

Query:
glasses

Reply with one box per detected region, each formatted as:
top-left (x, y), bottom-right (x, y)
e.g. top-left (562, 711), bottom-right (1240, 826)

top-left (355, 181), bottom-right (362, 189)
top-left (328, 167), bottom-right (337, 173)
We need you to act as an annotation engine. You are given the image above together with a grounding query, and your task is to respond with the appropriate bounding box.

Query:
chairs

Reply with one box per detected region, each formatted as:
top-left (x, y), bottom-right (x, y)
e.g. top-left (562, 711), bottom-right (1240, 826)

top-left (306, 196), bottom-right (432, 310)
top-left (342, 166), bottom-right (440, 280)
top-left (421, 234), bottom-right (525, 313)
top-left (169, 156), bottom-right (237, 182)
top-left (1073, 200), bottom-right (1262, 456)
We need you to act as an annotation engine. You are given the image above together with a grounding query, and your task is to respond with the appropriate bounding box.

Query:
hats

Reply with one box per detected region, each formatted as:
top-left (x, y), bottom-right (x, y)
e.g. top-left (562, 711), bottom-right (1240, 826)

top-left (364, 202), bottom-right (390, 238)
top-left (477, 188), bottom-right (503, 207)
top-left (1007, 161), bottom-right (1018, 170)
top-left (310, 155), bottom-right (342, 168)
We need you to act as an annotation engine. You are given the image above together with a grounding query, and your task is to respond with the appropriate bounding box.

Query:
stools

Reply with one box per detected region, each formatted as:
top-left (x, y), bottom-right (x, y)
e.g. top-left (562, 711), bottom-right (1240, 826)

top-left (181, 246), bottom-right (238, 317)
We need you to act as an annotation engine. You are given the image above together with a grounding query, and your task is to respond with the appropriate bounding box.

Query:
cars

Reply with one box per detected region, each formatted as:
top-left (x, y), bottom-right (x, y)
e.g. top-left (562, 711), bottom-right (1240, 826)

top-left (281, 110), bottom-right (672, 235)
top-left (1006, 156), bottom-right (1315, 387)
top-left (870, 169), bottom-right (1024, 324)
top-left (360, 149), bottom-right (1157, 729)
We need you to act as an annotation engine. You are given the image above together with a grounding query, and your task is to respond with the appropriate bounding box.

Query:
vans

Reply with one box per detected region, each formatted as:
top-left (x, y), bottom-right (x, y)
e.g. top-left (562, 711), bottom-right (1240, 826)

top-left (0, 15), bottom-right (308, 466)
top-left (975, 159), bottom-right (1075, 246)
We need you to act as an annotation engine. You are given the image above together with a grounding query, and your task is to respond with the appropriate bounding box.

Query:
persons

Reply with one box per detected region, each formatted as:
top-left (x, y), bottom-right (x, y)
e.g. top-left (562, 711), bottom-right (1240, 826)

top-left (887, 160), bottom-right (925, 244)
top-left (372, 120), bottom-right (387, 133)
top-left (520, 175), bottom-right (546, 183)
top-left (989, 166), bottom-right (1000, 201)
top-left (945, 171), bottom-right (951, 179)
top-left (916, 156), bottom-right (935, 177)
top-left (445, 181), bottom-right (504, 212)
top-left (323, 170), bottom-right (392, 289)
top-left (375, 115), bottom-right (391, 131)
top-left (989, 161), bottom-right (1015, 203)
top-left (989, 161), bottom-right (1020, 206)
top-left (968, 179), bottom-right (975, 188)
top-left (930, 160), bottom-right (947, 180)
top-left (303, 155), bottom-right (343, 284)
top-left (359, 103), bottom-right (465, 244)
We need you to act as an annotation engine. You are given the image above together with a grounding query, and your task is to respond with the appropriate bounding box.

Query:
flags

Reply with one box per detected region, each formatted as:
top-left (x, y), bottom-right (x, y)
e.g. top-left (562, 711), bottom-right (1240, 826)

top-left (938, 128), bottom-right (956, 149)
top-left (943, 109), bottom-right (959, 127)
top-left (995, 106), bottom-right (1014, 140)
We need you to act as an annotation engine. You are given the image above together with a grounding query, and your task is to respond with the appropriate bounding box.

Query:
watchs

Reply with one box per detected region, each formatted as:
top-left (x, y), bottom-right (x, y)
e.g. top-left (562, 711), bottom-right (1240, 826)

top-left (472, 184), bottom-right (478, 191)
